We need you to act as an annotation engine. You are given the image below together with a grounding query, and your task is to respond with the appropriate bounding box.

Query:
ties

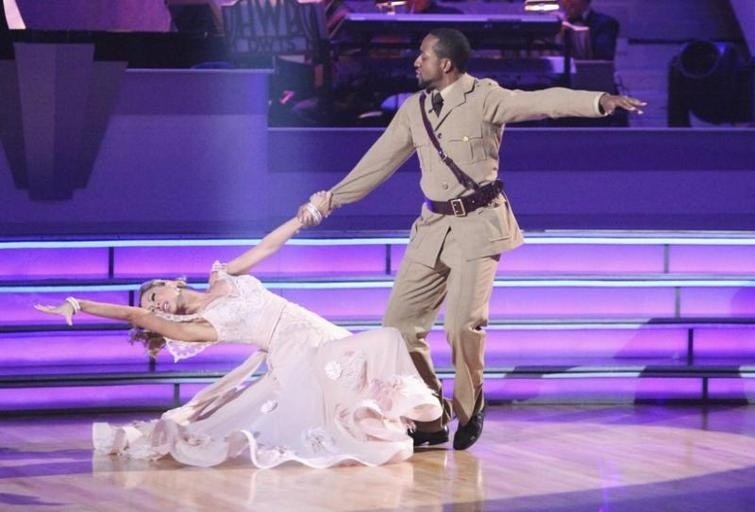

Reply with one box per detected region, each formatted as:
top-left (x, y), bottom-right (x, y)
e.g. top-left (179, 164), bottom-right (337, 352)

top-left (433, 92), bottom-right (442, 117)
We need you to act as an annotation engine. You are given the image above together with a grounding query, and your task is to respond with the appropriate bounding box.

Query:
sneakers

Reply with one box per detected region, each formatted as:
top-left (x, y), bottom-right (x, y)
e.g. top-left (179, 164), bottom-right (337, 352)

top-left (90, 421), bottom-right (141, 454)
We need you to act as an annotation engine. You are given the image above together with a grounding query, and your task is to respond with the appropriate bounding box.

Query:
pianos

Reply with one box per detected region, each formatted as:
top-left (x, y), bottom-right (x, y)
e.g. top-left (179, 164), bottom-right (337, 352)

top-left (329, 14), bottom-right (577, 87)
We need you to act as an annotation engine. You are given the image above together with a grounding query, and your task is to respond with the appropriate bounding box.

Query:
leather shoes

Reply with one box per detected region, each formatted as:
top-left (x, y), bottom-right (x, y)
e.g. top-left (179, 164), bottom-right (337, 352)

top-left (453, 392), bottom-right (487, 451)
top-left (408, 427), bottom-right (450, 446)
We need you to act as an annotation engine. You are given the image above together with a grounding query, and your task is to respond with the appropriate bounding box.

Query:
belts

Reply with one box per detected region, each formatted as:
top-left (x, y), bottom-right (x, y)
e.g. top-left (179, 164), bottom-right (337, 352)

top-left (424, 181), bottom-right (503, 216)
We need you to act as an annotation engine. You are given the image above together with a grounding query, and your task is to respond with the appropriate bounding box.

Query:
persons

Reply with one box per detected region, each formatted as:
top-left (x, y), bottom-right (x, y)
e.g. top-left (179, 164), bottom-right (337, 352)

top-left (296, 28), bottom-right (650, 451)
top-left (32, 189), bottom-right (444, 470)
top-left (668, 40), bottom-right (755, 129)
top-left (285, 1), bottom-right (467, 130)
top-left (557, 1), bottom-right (632, 128)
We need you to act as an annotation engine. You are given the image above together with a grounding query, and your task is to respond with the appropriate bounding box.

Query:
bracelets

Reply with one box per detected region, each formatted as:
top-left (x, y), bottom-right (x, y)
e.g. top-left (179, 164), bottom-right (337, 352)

top-left (307, 204), bottom-right (322, 226)
top-left (65, 296), bottom-right (80, 315)
